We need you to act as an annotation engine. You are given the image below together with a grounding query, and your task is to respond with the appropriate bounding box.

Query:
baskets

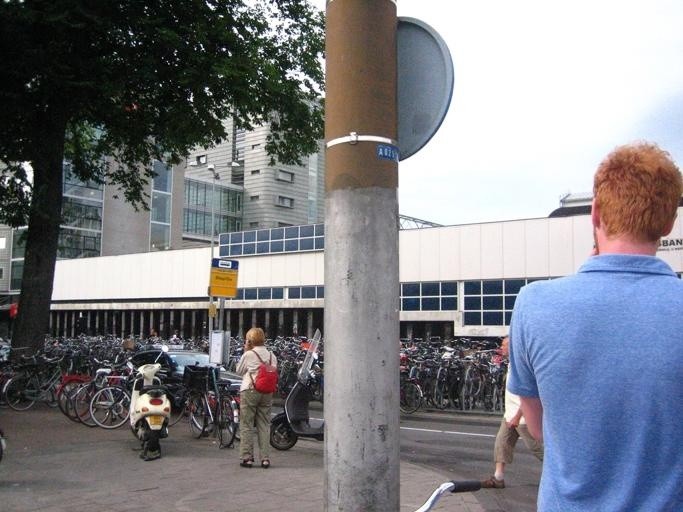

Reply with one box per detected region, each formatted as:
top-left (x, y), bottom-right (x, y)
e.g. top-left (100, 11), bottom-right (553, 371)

top-left (183, 365), bottom-right (208, 387)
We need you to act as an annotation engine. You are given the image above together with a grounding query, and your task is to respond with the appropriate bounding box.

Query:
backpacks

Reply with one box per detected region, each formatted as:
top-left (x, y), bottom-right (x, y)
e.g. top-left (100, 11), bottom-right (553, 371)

top-left (247, 350), bottom-right (279, 394)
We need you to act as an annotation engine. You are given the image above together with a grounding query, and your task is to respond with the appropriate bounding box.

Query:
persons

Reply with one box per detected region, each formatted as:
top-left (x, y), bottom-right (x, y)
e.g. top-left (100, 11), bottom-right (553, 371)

top-left (505, 139), bottom-right (683, 512)
top-left (480, 334), bottom-right (543, 491)
top-left (235, 327), bottom-right (278, 469)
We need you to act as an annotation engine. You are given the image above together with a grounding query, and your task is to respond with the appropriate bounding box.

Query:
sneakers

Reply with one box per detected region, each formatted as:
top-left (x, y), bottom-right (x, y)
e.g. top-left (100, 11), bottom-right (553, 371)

top-left (481, 476), bottom-right (505, 489)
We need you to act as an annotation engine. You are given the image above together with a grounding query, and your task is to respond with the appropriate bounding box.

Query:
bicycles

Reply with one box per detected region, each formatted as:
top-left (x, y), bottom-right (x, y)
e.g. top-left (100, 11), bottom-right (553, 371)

top-left (399, 336), bottom-right (510, 414)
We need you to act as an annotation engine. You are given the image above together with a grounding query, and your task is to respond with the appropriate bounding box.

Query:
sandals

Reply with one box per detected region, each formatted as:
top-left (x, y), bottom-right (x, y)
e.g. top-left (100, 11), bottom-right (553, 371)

top-left (261, 460), bottom-right (270, 468)
top-left (240, 459), bottom-right (252, 467)
